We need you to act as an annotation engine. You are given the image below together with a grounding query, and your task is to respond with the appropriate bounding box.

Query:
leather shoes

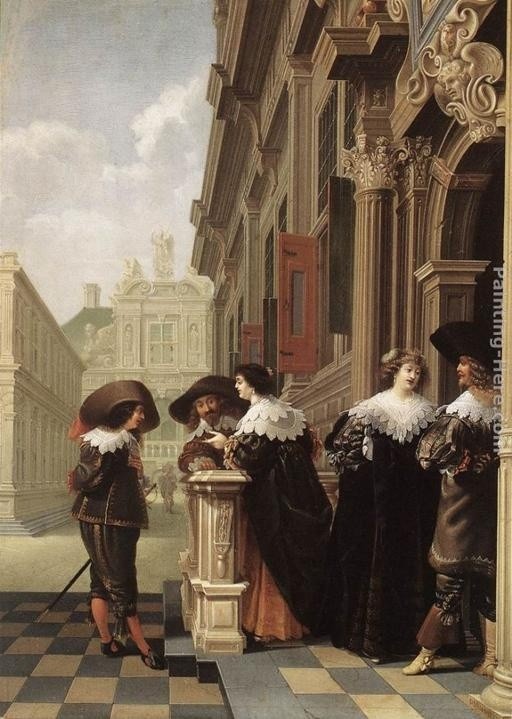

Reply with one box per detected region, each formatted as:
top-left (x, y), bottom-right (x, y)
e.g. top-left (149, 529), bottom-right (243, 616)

top-left (142, 651), bottom-right (167, 670)
top-left (362, 643), bottom-right (389, 664)
top-left (253, 634), bottom-right (277, 643)
top-left (100, 637), bottom-right (129, 658)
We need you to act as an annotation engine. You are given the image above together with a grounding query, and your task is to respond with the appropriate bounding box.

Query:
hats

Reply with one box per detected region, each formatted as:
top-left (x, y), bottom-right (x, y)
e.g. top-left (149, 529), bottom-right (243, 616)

top-left (429, 320), bottom-right (495, 361)
top-left (168, 376), bottom-right (250, 423)
top-left (80, 380), bottom-right (161, 435)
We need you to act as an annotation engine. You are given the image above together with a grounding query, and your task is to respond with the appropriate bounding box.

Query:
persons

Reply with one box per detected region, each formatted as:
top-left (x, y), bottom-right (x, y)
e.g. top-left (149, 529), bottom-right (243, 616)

top-left (159, 462), bottom-right (178, 514)
top-left (304, 349), bottom-right (443, 665)
top-left (401, 320), bottom-right (501, 676)
top-left (66, 380), bottom-right (166, 669)
top-left (201, 363), bottom-right (338, 649)
top-left (167, 373), bottom-right (251, 472)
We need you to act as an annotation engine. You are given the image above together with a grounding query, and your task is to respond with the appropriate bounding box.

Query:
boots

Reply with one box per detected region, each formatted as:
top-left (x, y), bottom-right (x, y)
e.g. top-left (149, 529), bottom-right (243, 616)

top-left (401, 650), bottom-right (436, 674)
